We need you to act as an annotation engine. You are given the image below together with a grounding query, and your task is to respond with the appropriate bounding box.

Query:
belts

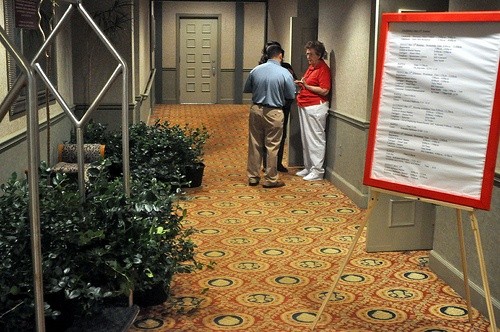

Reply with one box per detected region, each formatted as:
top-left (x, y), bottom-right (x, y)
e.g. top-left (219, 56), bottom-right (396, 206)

top-left (254, 103), bottom-right (282, 109)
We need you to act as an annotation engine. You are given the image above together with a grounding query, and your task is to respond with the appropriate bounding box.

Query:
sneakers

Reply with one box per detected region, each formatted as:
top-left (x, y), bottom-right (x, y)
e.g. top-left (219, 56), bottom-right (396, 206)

top-left (295, 169), bottom-right (309, 176)
top-left (303, 172), bottom-right (323, 180)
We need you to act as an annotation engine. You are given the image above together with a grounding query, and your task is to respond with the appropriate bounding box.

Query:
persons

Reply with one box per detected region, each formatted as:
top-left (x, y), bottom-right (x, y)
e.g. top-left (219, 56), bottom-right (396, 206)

top-left (243, 41), bottom-right (298, 189)
top-left (294, 40), bottom-right (331, 181)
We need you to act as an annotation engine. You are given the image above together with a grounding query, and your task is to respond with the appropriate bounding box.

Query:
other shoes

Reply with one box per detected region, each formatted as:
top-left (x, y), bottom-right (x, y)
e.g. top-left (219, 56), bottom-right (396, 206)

top-left (278, 167), bottom-right (288, 172)
top-left (263, 182), bottom-right (285, 188)
top-left (249, 179), bottom-right (259, 186)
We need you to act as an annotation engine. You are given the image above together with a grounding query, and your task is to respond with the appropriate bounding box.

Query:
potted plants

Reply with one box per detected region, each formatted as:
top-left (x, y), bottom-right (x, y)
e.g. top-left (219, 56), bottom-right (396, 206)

top-left (0, 118), bottom-right (217, 320)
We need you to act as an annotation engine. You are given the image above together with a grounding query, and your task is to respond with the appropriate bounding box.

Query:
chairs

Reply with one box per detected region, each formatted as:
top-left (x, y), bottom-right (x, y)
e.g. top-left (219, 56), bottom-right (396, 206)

top-left (45, 143), bottom-right (105, 188)
